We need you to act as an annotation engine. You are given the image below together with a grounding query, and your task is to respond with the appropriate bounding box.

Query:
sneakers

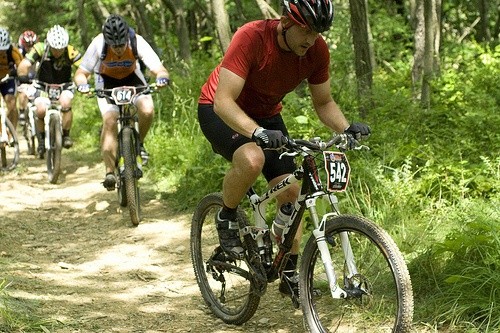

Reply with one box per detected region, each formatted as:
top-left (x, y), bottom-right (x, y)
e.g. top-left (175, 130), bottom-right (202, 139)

top-left (214, 207), bottom-right (246, 260)
top-left (279, 275), bottom-right (322, 300)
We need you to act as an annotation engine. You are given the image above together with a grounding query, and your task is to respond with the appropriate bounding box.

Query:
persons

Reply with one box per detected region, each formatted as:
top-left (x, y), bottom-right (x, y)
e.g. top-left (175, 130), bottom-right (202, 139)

top-left (198, 0), bottom-right (373, 307)
top-left (0, 15), bottom-right (170, 191)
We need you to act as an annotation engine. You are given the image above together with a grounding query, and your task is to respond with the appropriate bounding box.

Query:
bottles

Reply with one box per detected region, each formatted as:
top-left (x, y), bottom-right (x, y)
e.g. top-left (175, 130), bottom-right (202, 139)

top-left (271, 201), bottom-right (294, 241)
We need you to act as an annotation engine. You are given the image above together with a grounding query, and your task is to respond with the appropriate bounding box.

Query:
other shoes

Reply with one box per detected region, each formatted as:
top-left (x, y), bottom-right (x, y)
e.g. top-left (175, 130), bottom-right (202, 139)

top-left (140, 146), bottom-right (150, 166)
top-left (37, 139), bottom-right (46, 154)
top-left (63, 136), bottom-right (73, 147)
top-left (103, 174), bottom-right (117, 191)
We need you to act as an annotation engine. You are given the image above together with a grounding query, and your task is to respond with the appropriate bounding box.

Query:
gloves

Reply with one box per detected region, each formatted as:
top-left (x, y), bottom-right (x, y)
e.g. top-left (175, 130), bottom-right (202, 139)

top-left (19, 75), bottom-right (32, 84)
top-left (252, 127), bottom-right (289, 153)
top-left (77, 84), bottom-right (90, 92)
top-left (345, 123), bottom-right (372, 142)
top-left (156, 78), bottom-right (169, 87)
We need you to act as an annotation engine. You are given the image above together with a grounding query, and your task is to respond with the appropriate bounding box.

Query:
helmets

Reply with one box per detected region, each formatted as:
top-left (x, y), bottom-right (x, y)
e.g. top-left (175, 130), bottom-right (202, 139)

top-left (103, 14), bottom-right (130, 45)
top-left (19, 30), bottom-right (40, 49)
top-left (47, 24), bottom-right (69, 49)
top-left (0, 28), bottom-right (12, 50)
top-left (280, 0), bottom-right (334, 33)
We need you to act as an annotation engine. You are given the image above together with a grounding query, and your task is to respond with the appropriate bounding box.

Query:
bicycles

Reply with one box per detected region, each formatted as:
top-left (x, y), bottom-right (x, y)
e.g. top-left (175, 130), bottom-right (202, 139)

top-left (0, 62), bottom-right (41, 173)
top-left (28, 79), bottom-right (80, 185)
top-left (189, 130), bottom-right (414, 333)
top-left (84, 82), bottom-right (170, 225)
